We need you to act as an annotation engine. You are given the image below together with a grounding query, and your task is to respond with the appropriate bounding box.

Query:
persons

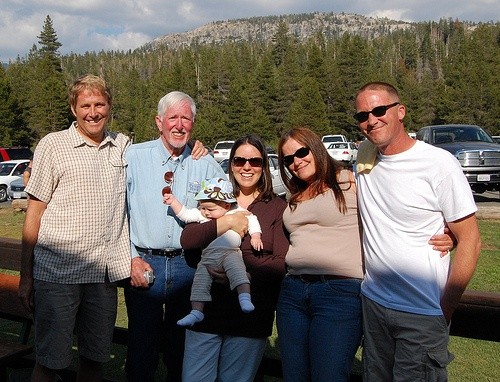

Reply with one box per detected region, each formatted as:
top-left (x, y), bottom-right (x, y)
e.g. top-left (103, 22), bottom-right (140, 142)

top-left (277, 126), bottom-right (458, 382)
top-left (18, 75), bottom-right (209, 382)
top-left (162, 177), bottom-right (263, 325)
top-left (181, 135), bottom-right (290, 382)
top-left (355, 82), bottom-right (481, 382)
top-left (124, 91), bottom-right (227, 382)
top-left (13, 155), bottom-right (34, 189)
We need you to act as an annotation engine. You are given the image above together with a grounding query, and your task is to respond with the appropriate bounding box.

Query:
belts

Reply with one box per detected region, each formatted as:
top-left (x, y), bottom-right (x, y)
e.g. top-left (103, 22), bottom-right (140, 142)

top-left (134, 246), bottom-right (183, 257)
top-left (288, 273), bottom-right (363, 283)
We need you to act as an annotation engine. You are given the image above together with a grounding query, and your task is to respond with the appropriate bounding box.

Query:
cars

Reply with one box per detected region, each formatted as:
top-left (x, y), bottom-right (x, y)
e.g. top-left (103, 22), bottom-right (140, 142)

top-left (321, 132), bottom-right (416, 168)
top-left (0, 147), bottom-right (34, 204)
top-left (416, 124), bottom-right (500, 193)
top-left (202, 141), bottom-right (293, 199)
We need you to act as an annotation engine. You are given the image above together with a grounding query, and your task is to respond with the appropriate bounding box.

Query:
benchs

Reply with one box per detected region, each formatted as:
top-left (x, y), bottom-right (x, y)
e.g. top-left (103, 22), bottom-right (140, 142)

top-left (1, 242), bottom-right (130, 382)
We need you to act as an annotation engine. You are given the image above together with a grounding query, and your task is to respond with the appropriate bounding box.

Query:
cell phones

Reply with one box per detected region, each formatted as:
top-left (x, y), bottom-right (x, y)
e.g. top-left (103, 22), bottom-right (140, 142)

top-left (144, 270), bottom-right (154, 284)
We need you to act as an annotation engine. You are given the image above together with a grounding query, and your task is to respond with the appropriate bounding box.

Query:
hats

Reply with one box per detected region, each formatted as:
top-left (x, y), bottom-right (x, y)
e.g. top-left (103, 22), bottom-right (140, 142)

top-left (193, 177), bottom-right (237, 204)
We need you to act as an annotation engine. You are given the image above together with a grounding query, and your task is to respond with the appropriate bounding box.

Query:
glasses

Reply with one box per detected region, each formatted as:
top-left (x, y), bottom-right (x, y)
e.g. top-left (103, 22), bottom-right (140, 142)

top-left (161, 171), bottom-right (175, 197)
top-left (282, 147), bottom-right (311, 166)
top-left (233, 156), bottom-right (263, 168)
top-left (352, 102), bottom-right (401, 123)
